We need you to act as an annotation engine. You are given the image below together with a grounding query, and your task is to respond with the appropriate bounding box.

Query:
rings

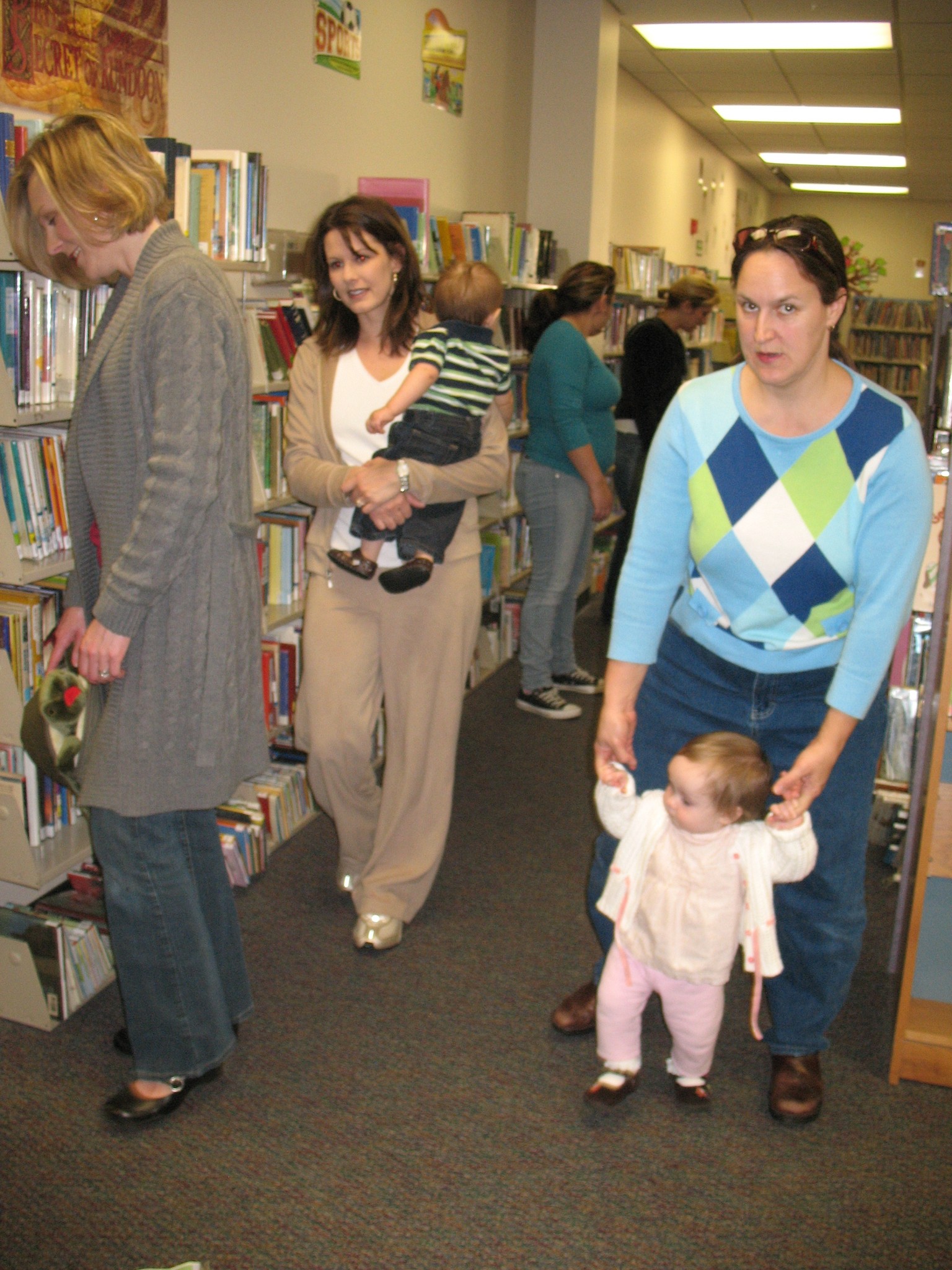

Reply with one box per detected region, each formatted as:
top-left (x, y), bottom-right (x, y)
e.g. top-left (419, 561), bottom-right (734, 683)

top-left (99, 670), bottom-right (109, 678)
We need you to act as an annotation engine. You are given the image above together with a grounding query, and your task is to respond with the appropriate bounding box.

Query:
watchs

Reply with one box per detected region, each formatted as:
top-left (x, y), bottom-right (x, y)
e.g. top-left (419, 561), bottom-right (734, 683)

top-left (397, 457), bottom-right (410, 493)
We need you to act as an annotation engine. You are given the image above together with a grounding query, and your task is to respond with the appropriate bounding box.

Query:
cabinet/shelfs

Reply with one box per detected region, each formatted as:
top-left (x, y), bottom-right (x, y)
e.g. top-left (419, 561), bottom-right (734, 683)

top-left (0, 281), bottom-right (952, 1033)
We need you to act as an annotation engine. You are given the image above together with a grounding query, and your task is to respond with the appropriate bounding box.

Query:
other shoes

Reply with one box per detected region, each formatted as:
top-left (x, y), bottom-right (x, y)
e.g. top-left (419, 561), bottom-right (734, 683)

top-left (766, 1051), bottom-right (827, 1127)
top-left (666, 1066), bottom-right (714, 1111)
top-left (550, 980), bottom-right (610, 1037)
top-left (105, 1060), bottom-right (222, 1135)
top-left (114, 1013), bottom-right (241, 1055)
top-left (327, 546), bottom-right (376, 579)
top-left (379, 557), bottom-right (433, 593)
top-left (333, 862), bottom-right (360, 898)
top-left (353, 908), bottom-right (405, 954)
top-left (582, 1066), bottom-right (637, 1114)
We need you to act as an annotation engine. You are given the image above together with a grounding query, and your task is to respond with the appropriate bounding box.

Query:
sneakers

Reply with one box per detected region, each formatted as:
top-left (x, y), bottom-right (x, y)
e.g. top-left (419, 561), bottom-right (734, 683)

top-left (513, 683), bottom-right (582, 718)
top-left (550, 665), bottom-right (605, 696)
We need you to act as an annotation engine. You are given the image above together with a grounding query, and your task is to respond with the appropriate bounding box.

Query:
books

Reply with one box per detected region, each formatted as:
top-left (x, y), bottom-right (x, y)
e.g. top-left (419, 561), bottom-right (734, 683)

top-left (0, 135), bottom-right (952, 1021)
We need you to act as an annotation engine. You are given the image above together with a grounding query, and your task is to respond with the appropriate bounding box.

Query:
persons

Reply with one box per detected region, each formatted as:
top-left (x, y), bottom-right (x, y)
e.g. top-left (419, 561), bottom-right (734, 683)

top-left (581, 731), bottom-right (819, 1112)
top-left (1, 106), bottom-right (272, 1130)
top-left (600, 275), bottom-right (721, 628)
top-left (548, 214), bottom-right (933, 1126)
top-left (514, 260), bottom-right (623, 721)
top-left (328, 257), bottom-right (515, 593)
top-left (282, 193), bottom-right (511, 953)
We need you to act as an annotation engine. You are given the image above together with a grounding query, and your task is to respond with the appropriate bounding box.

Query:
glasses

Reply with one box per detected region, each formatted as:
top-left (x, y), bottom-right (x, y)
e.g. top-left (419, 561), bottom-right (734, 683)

top-left (732, 225), bottom-right (841, 290)
